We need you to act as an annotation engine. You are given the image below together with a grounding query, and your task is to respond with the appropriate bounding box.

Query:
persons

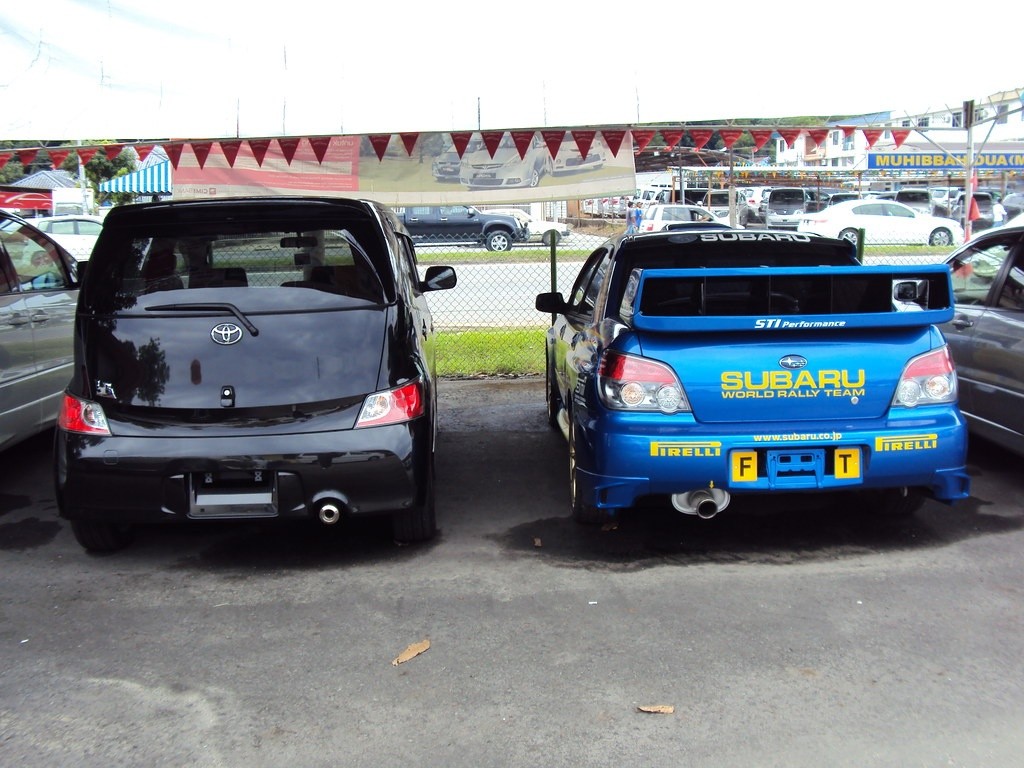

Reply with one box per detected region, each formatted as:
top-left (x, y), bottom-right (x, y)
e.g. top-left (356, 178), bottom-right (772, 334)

top-left (626, 200), bottom-right (644, 234)
top-left (698, 214), bottom-right (710, 223)
top-left (993, 199), bottom-right (1007, 228)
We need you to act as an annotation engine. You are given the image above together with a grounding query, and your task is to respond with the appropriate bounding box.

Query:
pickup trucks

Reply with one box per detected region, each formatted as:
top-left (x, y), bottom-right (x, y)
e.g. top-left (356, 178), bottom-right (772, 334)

top-left (396, 204), bottom-right (531, 253)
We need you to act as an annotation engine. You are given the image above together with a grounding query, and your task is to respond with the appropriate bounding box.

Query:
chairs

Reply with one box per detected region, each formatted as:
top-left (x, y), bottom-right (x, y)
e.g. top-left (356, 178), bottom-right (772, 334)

top-left (310, 265), bottom-right (355, 292)
top-left (187, 265), bottom-right (248, 288)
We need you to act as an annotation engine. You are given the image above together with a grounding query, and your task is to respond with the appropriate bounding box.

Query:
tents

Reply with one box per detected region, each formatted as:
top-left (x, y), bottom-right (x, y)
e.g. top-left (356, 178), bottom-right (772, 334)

top-left (99, 160), bottom-right (172, 196)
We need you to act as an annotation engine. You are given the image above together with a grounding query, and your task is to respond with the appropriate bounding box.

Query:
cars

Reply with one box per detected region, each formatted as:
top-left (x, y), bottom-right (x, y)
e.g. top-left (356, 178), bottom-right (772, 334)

top-left (480, 209), bottom-right (571, 246)
top-left (431, 140), bottom-right (485, 183)
top-left (0, 209), bottom-right (91, 454)
top-left (52, 196), bottom-right (459, 545)
top-left (535, 228), bottom-right (973, 526)
top-left (892, 224), bottom-right (1024, 460)
top-left (541, 131), bottom-right (605, 176)
top-left (582, 186), bottom-right (1024, 233)
top-left (2, 214), bottom-right (103, 270)
top-left (460, 134), bottom-right (545, 188)
top-left (638, 206), bottom-right (745, 234)
top-left (797, 200), bottom-right (965, 248)
